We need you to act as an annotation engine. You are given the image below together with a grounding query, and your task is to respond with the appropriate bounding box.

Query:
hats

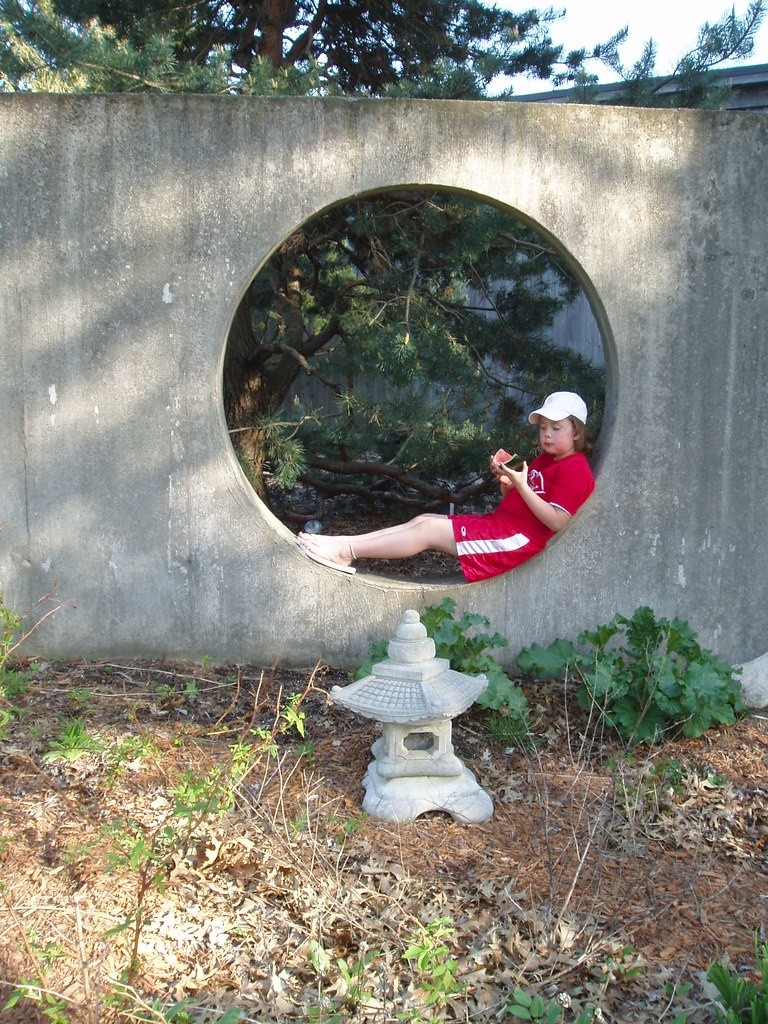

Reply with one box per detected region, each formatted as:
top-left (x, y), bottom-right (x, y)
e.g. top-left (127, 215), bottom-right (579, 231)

top-left (528, 391), bottom-right (587, 424)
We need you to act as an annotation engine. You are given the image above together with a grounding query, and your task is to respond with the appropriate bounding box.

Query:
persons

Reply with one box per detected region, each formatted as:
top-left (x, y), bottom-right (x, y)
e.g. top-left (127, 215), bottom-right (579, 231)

top-left (296, 392), bottom-right (594, 575)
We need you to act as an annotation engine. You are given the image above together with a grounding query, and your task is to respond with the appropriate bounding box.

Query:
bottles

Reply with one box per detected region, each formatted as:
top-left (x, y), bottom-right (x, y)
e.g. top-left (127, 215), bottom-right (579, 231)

top-left (304, 519), bottom-right (322, 534)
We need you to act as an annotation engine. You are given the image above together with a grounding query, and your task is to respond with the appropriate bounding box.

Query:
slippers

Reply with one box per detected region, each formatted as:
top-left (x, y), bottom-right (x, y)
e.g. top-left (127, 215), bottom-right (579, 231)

top-left (297, 531), bottom-right (357, 575)
